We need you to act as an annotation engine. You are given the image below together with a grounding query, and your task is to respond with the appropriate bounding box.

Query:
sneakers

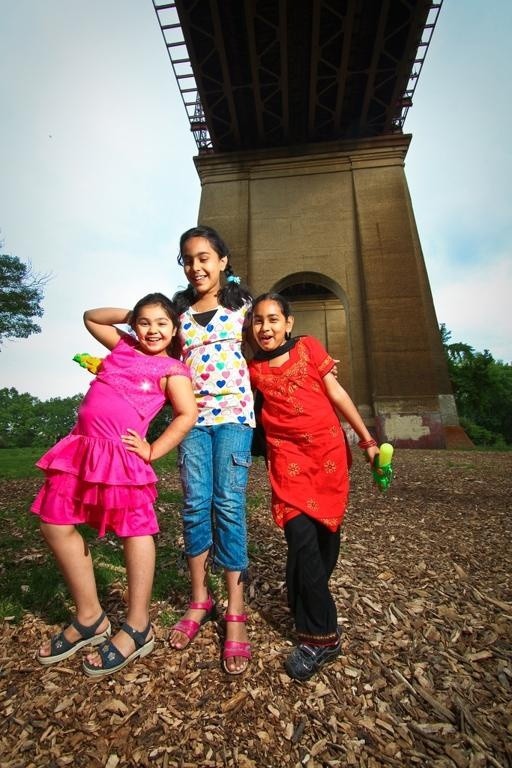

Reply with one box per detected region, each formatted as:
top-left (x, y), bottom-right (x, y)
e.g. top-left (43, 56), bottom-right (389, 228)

top-left (283, 639), bottom-right (340, 680)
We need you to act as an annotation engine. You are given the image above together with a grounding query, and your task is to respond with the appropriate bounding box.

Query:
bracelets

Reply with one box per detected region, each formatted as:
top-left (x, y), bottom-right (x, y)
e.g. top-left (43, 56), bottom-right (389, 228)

top-left (145, 444), bottom-right (155, 466)
top-left (358, 438), bottom-right (379, 449)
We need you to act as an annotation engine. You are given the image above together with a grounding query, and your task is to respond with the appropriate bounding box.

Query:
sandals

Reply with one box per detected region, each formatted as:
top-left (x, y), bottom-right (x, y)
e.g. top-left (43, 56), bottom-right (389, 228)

top-left (221, 612), bottom-right (252, 674)
top-left (81, 620), bottom-right (155, 680)
top-left (36, 611), bottom-right (112, 666)
top-left (167, 596), bottom-right (214, 650)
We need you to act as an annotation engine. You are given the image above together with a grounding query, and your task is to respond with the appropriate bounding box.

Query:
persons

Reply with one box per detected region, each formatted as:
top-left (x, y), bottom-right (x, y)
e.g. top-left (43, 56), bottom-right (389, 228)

top-left (31, 292), bottom-right (199, 678)
top-left (245, 292), bottom-right (385, 681)
top-left (169, 225), bottom-right (340, 675)
